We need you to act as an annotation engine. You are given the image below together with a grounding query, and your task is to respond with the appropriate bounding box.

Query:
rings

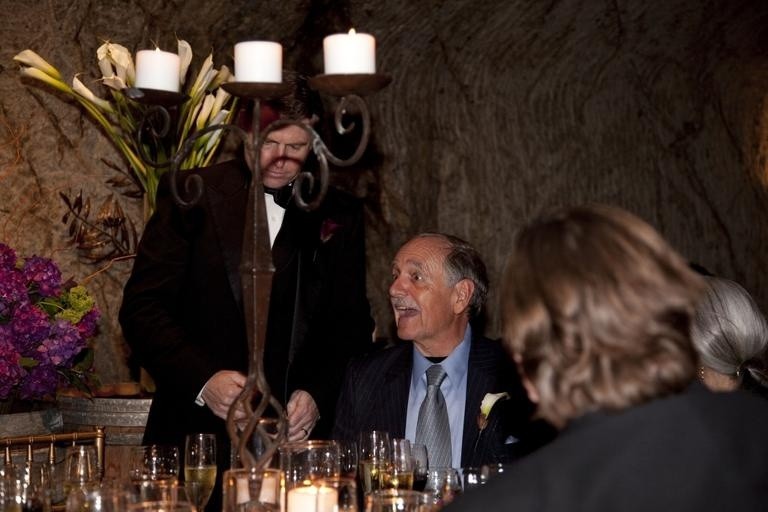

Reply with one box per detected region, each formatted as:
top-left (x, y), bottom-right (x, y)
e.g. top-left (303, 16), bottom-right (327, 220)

top-left (300, 428), bottom-right (308, 436)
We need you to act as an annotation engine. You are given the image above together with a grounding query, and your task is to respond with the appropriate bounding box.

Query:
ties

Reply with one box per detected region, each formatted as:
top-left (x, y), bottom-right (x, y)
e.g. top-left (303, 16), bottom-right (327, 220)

top-left (414, 364), bottom-right (453, 489)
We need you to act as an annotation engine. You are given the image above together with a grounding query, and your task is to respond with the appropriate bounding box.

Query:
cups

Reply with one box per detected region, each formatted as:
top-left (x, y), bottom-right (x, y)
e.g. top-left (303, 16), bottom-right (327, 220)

top-left (0, 421), bottom-right (496, 512)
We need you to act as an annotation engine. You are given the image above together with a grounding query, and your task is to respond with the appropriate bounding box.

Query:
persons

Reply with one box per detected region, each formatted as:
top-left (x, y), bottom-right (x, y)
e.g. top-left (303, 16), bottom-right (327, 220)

top-left (687, 275), bottom-right (768, 394)
top-left (328, 230), bottom-right (558, 496)
top-left (118, 66), bottom-right (377, 511)
top-left (434, 198), bottom-right (768, 511)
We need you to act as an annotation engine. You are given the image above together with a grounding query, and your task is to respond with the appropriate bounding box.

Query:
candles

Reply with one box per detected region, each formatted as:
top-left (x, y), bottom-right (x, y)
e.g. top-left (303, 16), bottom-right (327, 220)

top-left (135, 27), bottom-right (377, 92)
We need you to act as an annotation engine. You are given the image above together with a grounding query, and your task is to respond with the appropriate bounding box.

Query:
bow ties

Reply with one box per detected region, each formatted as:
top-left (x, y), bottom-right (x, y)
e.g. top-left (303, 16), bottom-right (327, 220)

top-left (263, 187), bottom-right (292, 209)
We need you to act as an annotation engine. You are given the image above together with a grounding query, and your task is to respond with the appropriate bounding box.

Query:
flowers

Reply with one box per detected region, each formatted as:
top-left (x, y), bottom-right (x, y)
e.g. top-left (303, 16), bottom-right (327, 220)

top-left (13, 31), bottom-right (240, 221)
top-left (1, 243), bottom-right (104, 402)
